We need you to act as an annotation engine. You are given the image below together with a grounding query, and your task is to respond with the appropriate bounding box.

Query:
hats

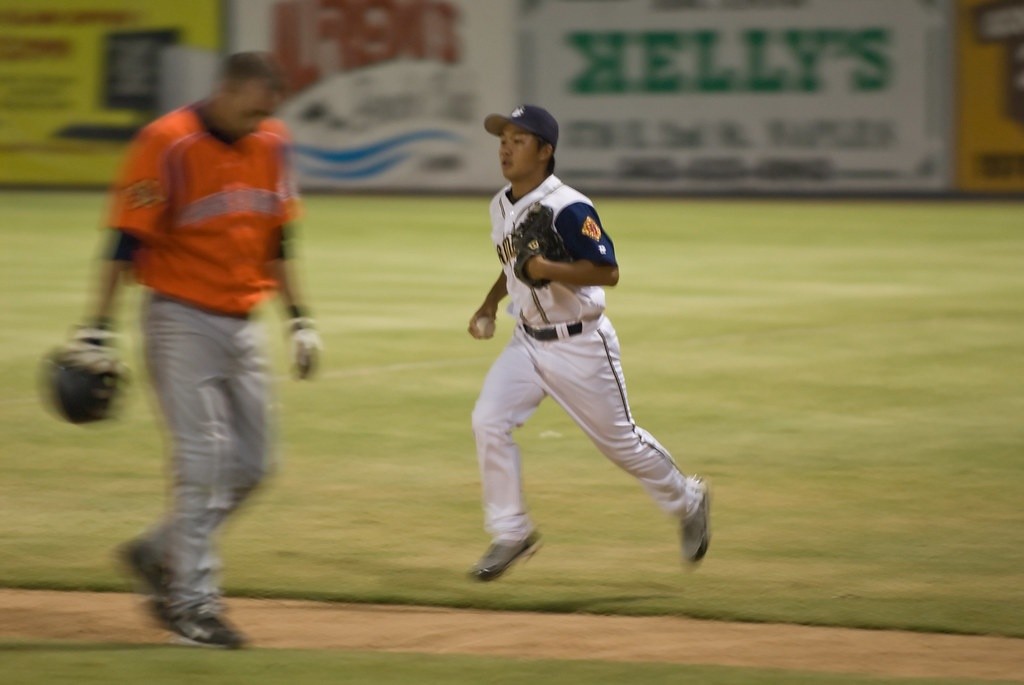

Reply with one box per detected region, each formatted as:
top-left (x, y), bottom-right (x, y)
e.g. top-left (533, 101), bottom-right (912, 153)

top-left (484, 103), bottom-right (559, 148)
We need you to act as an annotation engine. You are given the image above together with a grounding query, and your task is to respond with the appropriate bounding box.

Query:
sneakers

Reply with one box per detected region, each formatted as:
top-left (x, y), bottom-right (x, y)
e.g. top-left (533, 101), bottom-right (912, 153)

top-left (147, 593), bottom-right (250, 648)
top-left (119, 537), bottom-right (170, 596)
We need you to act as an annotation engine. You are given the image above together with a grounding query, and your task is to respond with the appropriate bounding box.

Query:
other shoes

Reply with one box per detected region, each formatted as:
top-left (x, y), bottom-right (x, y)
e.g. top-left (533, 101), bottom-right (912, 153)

top-left (680, 478), bottom-right (712, 565)
top-left (467, 530), bottom-right (545, 584)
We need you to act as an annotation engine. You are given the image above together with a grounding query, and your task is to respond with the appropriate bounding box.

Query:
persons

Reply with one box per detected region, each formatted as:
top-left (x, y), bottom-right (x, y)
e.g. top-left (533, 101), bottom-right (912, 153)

top-left (468, 103), bottom-right (712, 581)
top-left (41, 50), bottom-right (321, 650)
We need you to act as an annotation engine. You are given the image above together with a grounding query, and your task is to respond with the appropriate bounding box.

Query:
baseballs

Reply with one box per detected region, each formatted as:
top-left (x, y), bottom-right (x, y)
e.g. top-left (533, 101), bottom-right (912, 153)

top-left (476, 317), bottom-right (496, 337)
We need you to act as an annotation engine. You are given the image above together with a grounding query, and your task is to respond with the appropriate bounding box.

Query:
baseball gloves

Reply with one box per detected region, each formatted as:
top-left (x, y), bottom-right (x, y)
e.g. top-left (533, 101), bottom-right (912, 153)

top-left (510, 200), bottom-right (564, 289)
top-left (36, 340), bottom-right (134, 426)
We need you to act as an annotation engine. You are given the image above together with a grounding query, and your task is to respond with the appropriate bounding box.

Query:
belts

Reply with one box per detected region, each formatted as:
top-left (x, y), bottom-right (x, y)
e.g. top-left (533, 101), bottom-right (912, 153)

top-left (519, 320), bottom-right (583, 340)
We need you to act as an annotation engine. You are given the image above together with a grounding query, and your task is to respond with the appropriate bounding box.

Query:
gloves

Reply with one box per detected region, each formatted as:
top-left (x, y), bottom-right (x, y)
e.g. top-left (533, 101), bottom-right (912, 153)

top-left (285, 304), bottom-right (323, 382)
